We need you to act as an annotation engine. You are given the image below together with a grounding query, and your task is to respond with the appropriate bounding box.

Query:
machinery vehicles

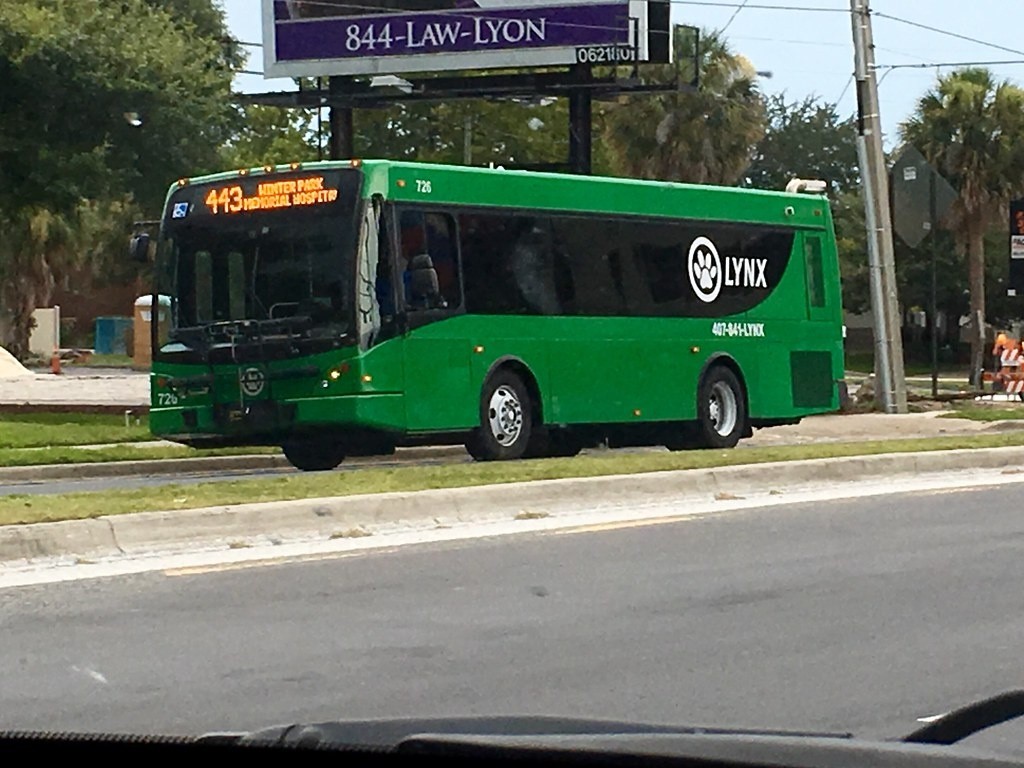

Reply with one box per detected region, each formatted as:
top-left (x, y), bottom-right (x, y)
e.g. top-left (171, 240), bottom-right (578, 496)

top-left (974, 321), bottom-right (1024, 402)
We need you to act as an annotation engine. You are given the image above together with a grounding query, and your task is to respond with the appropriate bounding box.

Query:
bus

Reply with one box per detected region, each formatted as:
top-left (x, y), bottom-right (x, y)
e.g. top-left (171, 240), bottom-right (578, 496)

top-left (130, 158), bottom-right (851, 471)
